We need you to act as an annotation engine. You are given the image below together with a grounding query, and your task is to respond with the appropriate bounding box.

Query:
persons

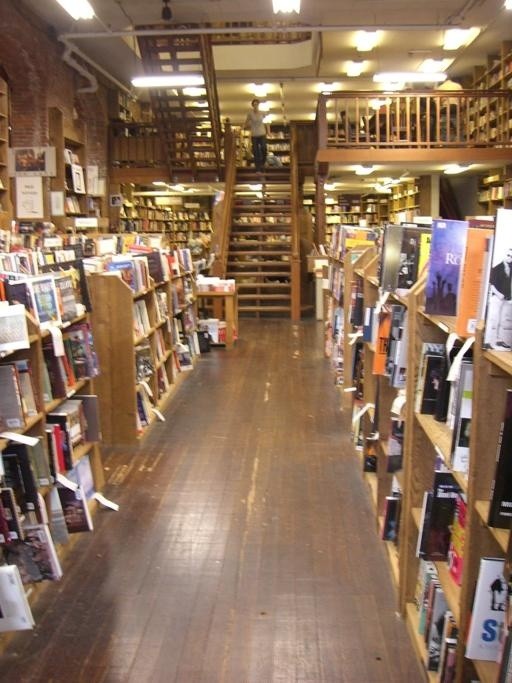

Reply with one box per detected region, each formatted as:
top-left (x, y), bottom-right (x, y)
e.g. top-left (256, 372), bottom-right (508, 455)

top-left (243, 99), bottom-right (273, 175)
top-left (267, 151), bottom-right (283, 166)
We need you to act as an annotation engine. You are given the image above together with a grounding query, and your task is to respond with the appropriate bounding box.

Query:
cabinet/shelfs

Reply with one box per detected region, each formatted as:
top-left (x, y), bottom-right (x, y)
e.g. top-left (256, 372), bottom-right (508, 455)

top-left (318, 191), bottom-right (421, 233)
top-left (243, 123), bottom-right (293, 166)
top-left (459, 41), bottom-right (511, 146)
top-left (339, 238), bottom-right (417, 613)
top-left (1, 77), bottom-right (17, 237)
top-left (317, 242), bottom-right (350, 408)
top-left (50, 111), bottom-right (92, 230)
top-left (121, 195), bottom-right (213, 246)
top-left (395, 262), bottom-right (511, 683)
top-left (180, 122), bottom-right (241, 169)
top-left (0, 267), bottom-right (118, 656)
top-left (78, 248), bottom-right (201, 451)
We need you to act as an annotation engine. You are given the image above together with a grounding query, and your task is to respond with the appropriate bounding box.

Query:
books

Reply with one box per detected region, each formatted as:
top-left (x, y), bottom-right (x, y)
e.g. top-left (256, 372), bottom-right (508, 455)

top-left (239, 216), bottom-right (291, 283)
top-left (3, 146), bottom-right (239, 634)
top-left (310, 206), bottom-right (512, 681)
top-left (465, 63), bottom-right (512, 147)
top-left (266, 132), bottom-right (290, 163)
top-left (303, 167), bottom-right (512, 242)
top-left (119, 93), bottom-right (250, 166)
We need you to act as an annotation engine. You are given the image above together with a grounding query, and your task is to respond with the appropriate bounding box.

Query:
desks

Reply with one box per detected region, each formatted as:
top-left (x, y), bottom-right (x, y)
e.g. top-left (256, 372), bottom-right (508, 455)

top-left (192, 287), bottom-right (238, 352)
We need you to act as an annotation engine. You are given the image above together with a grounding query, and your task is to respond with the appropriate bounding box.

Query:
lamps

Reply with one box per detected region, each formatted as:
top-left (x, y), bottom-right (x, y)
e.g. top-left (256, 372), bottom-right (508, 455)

top-left (248, 185), bottom-right (271, 199)
top-left (241, 5), bottom-right (461, 123)
top-left (50, 0), bottom-right (102, 21)
top-left (161, 182), bottom-right (203, 195)
top-left (270, 0), bottom-right (306, 18)
top-left (129, 69), bottom-right (207, 91)
top-left (324, 177), bottom-right (401, 195)
